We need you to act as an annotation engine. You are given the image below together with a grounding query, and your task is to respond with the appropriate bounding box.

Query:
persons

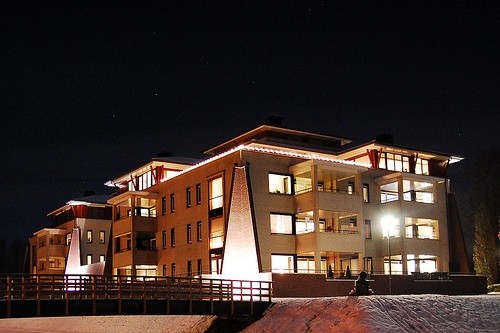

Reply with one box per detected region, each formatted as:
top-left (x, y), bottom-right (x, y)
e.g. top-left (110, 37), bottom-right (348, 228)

top-left (351, 271), bottom-right (373, 294)
